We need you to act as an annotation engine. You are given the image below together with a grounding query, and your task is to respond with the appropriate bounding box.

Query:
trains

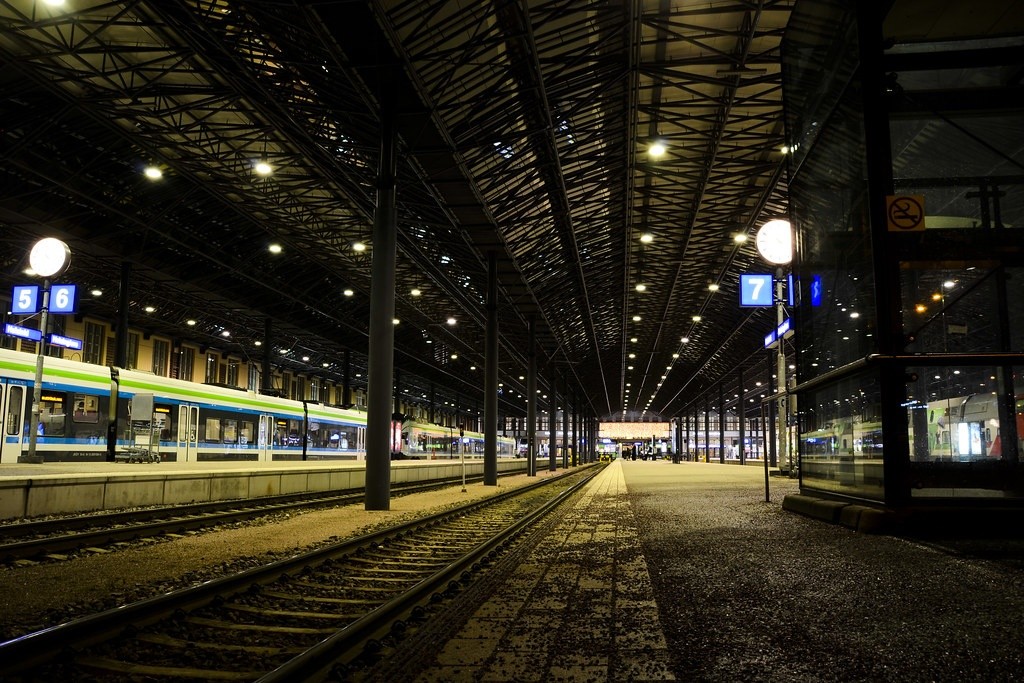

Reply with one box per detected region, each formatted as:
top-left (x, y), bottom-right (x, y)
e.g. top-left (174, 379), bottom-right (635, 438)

top-left (0, 346), bottom-right (518, 464)
top-left (802, 388), bottom-right (1024, 484)
